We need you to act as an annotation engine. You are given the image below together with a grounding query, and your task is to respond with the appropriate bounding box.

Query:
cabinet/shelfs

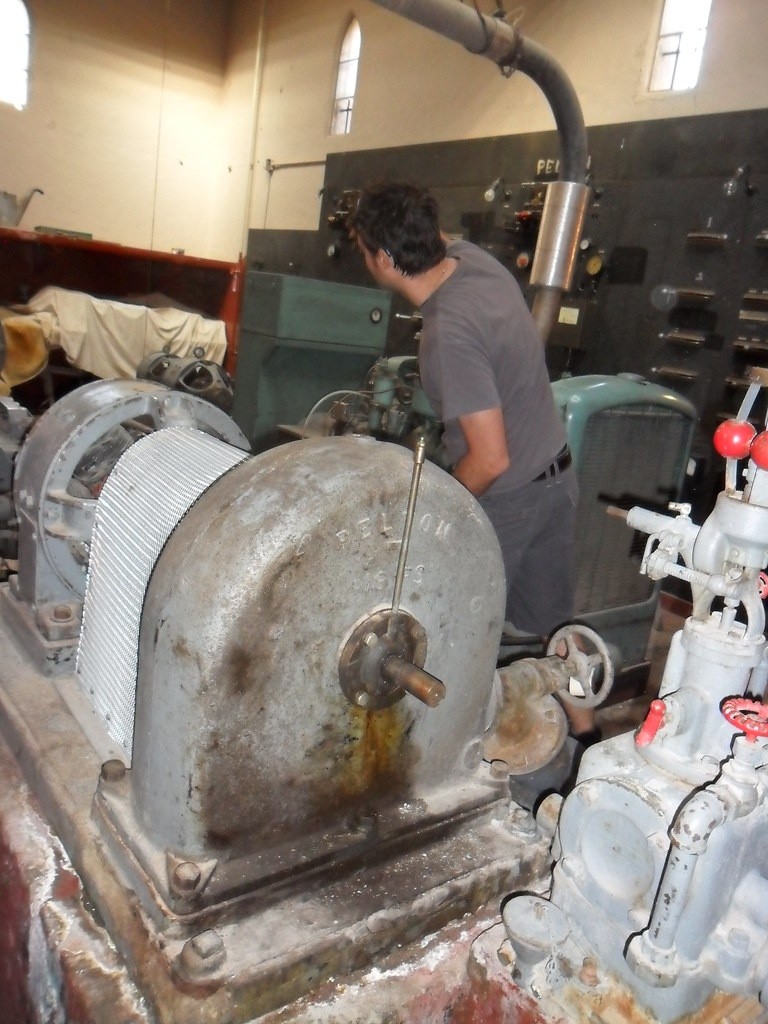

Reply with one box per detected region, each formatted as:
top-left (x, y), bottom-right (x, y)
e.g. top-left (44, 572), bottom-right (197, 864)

top-left (1, 224), bottom-right (246, 418)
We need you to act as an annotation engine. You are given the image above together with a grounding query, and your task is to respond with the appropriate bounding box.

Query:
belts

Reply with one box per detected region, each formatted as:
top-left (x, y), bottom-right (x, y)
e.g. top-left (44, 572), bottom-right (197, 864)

top-left (531, 444), bottom-right (573, 483)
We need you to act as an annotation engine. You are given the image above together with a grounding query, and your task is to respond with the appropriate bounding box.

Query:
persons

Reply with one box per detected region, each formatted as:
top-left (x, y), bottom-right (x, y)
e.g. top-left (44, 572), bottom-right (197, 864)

top-left (350, 174), bottom-right (580, 634)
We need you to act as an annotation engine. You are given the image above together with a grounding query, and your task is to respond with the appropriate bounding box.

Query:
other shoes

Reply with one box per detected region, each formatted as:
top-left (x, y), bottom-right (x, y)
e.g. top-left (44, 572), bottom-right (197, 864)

top-left (566, 725), bottom-right (606, 748)
